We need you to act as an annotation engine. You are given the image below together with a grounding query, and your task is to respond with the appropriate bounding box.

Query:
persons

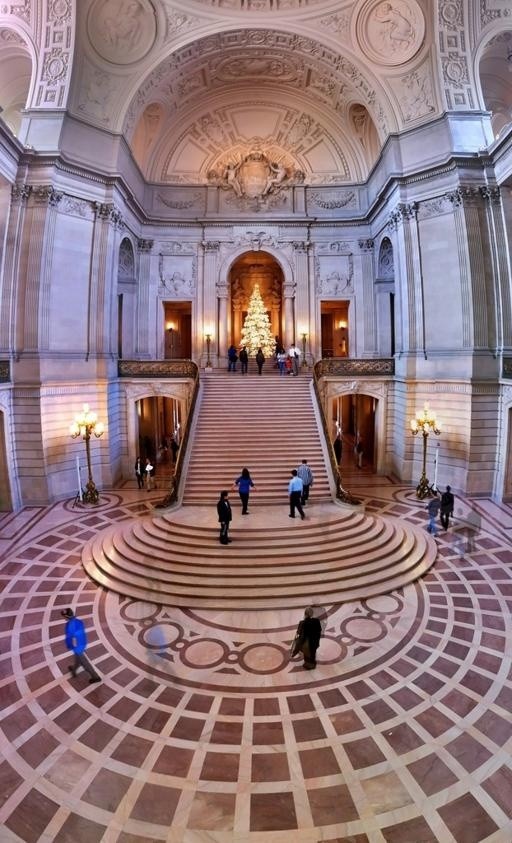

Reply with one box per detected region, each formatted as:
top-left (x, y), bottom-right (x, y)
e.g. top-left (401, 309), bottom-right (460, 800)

top-left (223, 160), bottom-right (243, 198)
top-left (374, 3), bottom-right (415, 51)
top-left (288, 469), bottom-right (305, 521)
top-left (334, 436), bottom-right (342, 464)
top-left (135, 456), bottom-right (144, 489)
top-left (231, 468), bottom-right (257, 516)
top-left (427, 491), bottom-right (442, 537)
top-left (104, 2), bottom-right (142, 50)
top-left (144, 458), bottom-right (157, 492)
top-left (440, 485), bottom-right (454, 532)
top-left (171, 439), bottom-right (178, 464)
top-left (227, 340), bottom-right (301, 376)
top-left (357, 437), bottom-right (364, 469)
top-left (262, 161), bottom-right (285, 196)
top-left (292, 607), bottom-right (322, 671)
top-left (296, 460), bottom-right (313, 505)
top-left (60, 608), bottom-right (101, 683)
top-left (217, 490), bottom-right (232, 544)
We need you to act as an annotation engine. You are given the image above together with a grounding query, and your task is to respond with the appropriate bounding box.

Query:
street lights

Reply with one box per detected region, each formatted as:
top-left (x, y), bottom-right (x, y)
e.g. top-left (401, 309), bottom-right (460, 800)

top-left (69, 401), bottom-right (105, 505)
top-left (335, 318), bottom-right (348, 357)
top-left (166, 319), bottom-right (179, 359)
top-left (408, 398), bottom-right (445, 500)
top-left (202, 325), bottom-right (215, 373)
top-left (299, 323), bottom-right (311, 373)
